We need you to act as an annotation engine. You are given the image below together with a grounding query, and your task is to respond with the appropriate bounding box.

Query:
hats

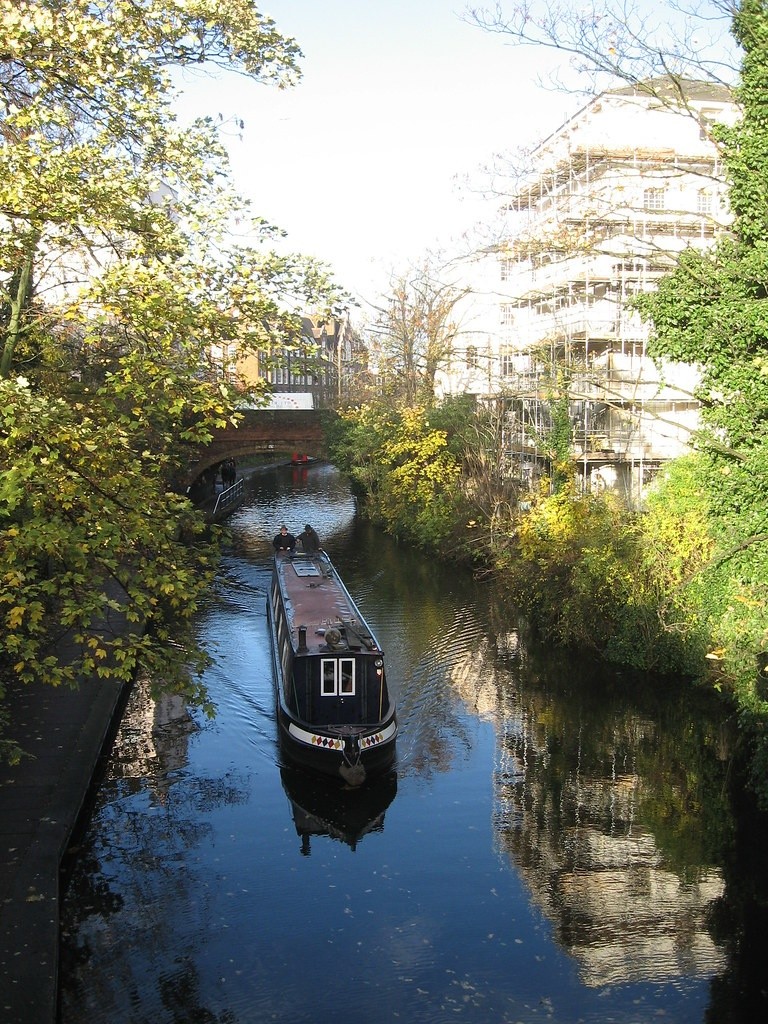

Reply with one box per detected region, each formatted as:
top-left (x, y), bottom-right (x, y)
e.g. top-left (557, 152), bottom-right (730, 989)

top-left (304, 524), bottom-right (313, 529)
top-left (280, 525), bottom-right (288, 531)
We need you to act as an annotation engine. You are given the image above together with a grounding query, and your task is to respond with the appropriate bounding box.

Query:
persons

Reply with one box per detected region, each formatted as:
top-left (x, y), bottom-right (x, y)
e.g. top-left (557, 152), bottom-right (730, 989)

top-left (296, 525), bottom-right (322, 552)
top-left (221, 460), bottom-right (236, 490)
top-left (273, 525), bottom-right (296, 551)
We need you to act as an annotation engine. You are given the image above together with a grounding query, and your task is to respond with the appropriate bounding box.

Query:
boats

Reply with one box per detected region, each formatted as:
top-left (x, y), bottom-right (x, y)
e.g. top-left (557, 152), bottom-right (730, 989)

top-left (266, 546), bottom-right (399, 753)
